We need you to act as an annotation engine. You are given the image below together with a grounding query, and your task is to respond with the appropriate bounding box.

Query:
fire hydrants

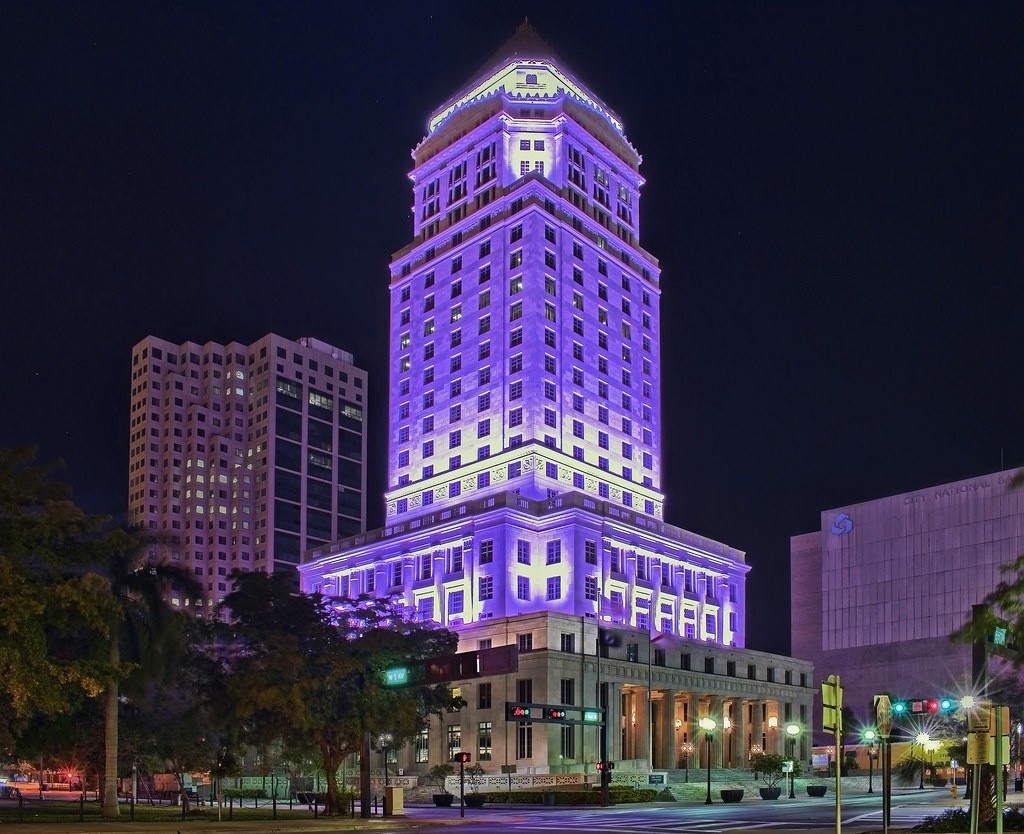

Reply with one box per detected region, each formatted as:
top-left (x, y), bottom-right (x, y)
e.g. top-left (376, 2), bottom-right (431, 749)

top-left (949, 783), bottom-right (959, 800)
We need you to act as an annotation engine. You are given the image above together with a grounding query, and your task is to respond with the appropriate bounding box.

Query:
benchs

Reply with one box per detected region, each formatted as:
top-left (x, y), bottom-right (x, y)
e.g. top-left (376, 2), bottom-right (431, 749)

top-left (147, 790), bottom-right (191, 805)
top-left (117, 792), bottom-right (132, 803)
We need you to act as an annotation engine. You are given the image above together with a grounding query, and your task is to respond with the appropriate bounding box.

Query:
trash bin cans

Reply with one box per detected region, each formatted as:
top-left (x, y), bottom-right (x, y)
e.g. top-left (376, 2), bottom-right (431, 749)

top-left (171, 794), bottom-right (182, 806)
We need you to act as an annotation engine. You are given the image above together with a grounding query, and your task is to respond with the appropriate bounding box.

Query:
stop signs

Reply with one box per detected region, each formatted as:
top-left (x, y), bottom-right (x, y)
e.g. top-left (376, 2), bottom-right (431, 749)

top-left (876, 695), bottom-right (893, 740)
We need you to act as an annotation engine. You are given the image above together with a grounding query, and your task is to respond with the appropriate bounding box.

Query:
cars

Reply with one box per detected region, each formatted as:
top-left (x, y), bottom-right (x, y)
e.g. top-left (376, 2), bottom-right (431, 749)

top-left (40, 785), bottom-right (49, 792)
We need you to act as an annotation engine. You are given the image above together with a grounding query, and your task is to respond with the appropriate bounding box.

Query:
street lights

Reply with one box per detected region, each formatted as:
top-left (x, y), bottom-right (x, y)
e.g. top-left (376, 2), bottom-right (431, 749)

top-left (864, 729), bottom-right (876, 794)
top-left (787, 725), bottom-right (800, 798)
top-left (702, 718), bottom-right (717, 803)
top-left (916, 733), bottom-right (929, 789)
top-left (824, 747), bottom-right (836, 777)
top-left (379, 733), bottom-right (392, 785)
top-left (680, 743), bottom-right (695, 783)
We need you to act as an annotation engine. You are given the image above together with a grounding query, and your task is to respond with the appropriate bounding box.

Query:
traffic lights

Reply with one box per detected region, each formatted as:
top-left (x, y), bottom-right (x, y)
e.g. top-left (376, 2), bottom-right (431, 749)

top-left (609, 763), bottom-right (615, 770)
top-left (894, 702), bottom-right (907, 712)
top-left (598, 763), bottom-right (604, 770)
top-left (455, 752), bottom-right (471, 763)
top-left (549, 708), bottom-right (566, 720)
top-left (927, 700), bottom-right (954, 711)
top-left (511, 706), bottom-right (530, 718)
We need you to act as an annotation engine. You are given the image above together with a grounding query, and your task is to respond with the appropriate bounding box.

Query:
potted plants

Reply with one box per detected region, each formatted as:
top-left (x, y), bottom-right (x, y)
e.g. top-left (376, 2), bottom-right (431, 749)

top-left (425, 763), bottom-right (456, 806)
top-left (464, 762), bottom-right (487, 806)
top-left (750, 751), bottom-right (783, 799)
top-left (297, 791), bottom-right (314, 803)
top-left (542, 787), bottom-right (557, 806)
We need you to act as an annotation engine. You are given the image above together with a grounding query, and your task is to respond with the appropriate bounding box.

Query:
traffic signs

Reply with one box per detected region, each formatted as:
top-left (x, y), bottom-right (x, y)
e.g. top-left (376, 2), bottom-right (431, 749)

top-left (966, 700), bottom-right (992, 732)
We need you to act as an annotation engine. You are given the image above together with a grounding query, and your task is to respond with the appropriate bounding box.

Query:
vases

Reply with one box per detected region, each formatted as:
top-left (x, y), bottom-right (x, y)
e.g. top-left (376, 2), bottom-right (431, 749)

top-left (932, 778), bottom-right (947, 788)
top-left (807, 785), bottom-right (828, 797)
top-left (951, 778), bottom-right (966, 785)
top-left (721, 790), bottom-right (745, 802)
